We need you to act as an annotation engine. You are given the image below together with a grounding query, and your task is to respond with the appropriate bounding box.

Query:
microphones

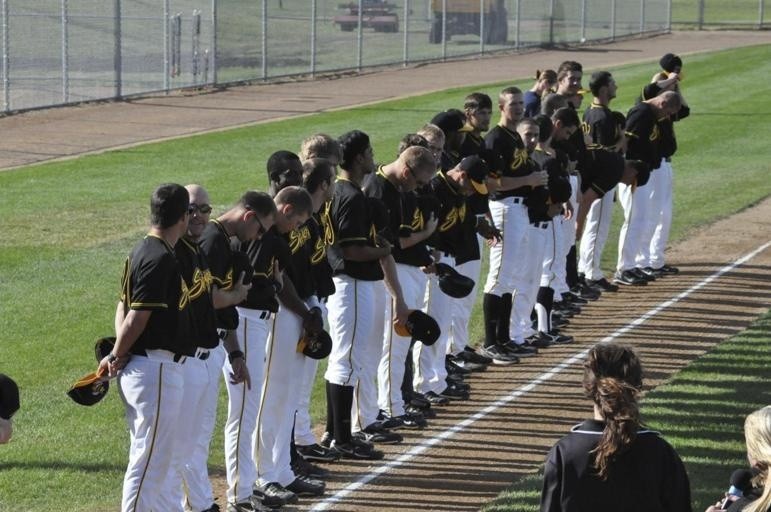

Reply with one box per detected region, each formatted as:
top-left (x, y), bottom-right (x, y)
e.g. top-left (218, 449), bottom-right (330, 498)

top-left (720, 469), bottom-right (749, 509)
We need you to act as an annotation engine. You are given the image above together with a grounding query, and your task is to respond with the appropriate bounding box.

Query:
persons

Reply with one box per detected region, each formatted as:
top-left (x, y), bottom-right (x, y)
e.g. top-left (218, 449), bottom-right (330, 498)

top-left (704, 406), bottom-right (771, 512)
top-left (0, 373), bottom-right (20, 444)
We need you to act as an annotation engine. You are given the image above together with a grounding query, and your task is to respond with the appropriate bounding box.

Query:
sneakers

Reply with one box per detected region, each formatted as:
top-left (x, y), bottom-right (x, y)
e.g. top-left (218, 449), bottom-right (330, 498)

top-left (532, 221), bottom-right (549, 229)
top-left (201, 445), bottom-right (341, 512)
top-left (320, 264), bottom-right (679, 462)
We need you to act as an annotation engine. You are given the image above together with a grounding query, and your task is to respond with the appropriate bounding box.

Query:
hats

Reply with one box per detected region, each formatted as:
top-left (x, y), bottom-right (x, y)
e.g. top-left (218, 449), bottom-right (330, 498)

top-left (417, 194), bottom-right (443, 230)
top-left (660, 53), bottom-right (686, 80)
top-left (94, 337), bottom-right (120, 365)
top-left (436, 262), bottom-right (475, 298)
top-left (543, 145), bottom-right (568, 169)
top-left (461, 155), bottom-right (490, 195)
top-left (541, 158), bottom-right (572, 205)
top-left (394, 310), bottom-right (441, 344)
top-left (631, 160), bottom-right (650, 194)
top-left (66, 365), bottom-right (109, 405)
top-left (295, 327), bottom-right (332, 359)
top-left (366, 195), bottom-right (390, 248)
top-left (576, 85), bottom-right (591, 95)
top-left (431, 111), bottom-right (474, 132)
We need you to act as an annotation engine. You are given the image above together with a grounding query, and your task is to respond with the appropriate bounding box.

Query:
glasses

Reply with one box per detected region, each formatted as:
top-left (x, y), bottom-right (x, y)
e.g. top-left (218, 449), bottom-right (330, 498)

top-left (188, 203), bottom-right (211, 214)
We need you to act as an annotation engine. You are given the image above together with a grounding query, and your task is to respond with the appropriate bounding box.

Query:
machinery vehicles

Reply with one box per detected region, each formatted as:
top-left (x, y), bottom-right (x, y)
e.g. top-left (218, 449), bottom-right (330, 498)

top-left (335, 0), bottom-right (400, 33)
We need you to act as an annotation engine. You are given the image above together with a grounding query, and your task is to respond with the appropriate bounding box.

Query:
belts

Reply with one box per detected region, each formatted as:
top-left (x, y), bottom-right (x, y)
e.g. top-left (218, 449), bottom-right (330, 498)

top-left (132, 346), bottom-right (186, 365)
top-left (514, 196), bottom-right (529, 205)
top-left (186, 345), bottom-right (210, 361)
top-left (260, 311), bottom-right (272, 320)
top-left (216, 328), bottom-right (228, 340)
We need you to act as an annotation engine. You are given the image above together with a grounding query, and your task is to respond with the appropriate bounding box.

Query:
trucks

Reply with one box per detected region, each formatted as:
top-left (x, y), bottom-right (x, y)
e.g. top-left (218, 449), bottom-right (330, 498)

top-left (428, 0), bottom-right (509, 46)
